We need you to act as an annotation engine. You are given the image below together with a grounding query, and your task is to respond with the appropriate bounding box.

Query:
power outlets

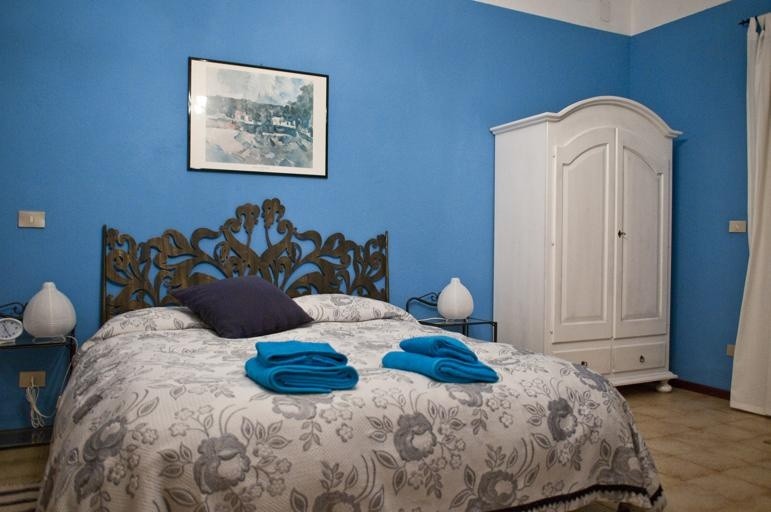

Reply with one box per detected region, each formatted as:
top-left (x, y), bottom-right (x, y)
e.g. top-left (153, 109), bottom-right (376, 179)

top-left (19, 371), bottom-right (46, 388)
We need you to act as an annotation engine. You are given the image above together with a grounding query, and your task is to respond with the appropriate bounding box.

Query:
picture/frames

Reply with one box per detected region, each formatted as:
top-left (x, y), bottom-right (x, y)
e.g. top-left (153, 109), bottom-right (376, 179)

top-left (187, 56), bottom-right (328, 179)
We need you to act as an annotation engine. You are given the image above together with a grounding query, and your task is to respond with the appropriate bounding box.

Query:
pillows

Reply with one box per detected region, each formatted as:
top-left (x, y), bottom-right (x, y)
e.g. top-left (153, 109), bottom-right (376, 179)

top-left (170, 276), bottom-right (314, 338)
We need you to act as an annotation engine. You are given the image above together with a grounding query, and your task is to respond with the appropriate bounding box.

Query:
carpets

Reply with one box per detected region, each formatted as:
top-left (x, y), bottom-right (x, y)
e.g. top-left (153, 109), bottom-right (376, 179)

top-left (0, 480), bottom-right (40, 512)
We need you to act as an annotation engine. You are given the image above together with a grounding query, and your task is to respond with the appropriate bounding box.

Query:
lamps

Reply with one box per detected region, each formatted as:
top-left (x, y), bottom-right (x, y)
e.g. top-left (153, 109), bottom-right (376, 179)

top-left (23, 282), bottom-right (76, 343)
top-left (438, 279), bottom-right (474, 322)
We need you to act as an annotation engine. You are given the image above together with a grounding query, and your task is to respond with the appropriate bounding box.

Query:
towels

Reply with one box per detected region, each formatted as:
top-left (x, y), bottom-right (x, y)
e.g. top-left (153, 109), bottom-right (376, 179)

top-left (245, 356), bottom-right (359, 393)
top-left (255, 341), bottom-right (348, 367)
top-left (399, 335), bottom-right (479, 363)
top-left (382, 351), bottom-right (498, 383)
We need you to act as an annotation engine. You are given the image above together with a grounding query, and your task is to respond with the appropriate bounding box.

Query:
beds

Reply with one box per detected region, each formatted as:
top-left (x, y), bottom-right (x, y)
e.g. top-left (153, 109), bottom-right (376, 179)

top-left (37, 198), bottom-right (668, 512)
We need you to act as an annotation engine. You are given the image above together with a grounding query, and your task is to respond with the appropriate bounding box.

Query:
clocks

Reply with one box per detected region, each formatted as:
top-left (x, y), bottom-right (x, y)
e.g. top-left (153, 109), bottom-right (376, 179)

top-left (0, 317), bottom-right (24, 343)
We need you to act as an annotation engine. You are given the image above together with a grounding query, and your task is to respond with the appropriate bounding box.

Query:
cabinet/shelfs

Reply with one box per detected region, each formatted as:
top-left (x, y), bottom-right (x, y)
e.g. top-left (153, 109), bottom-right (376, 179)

top-left (489, 95), bottom-right (683, 392)
top-left (0, 302), bottom-right (79, 450)
top-left (406, 292), bottom-right (497, 343)
